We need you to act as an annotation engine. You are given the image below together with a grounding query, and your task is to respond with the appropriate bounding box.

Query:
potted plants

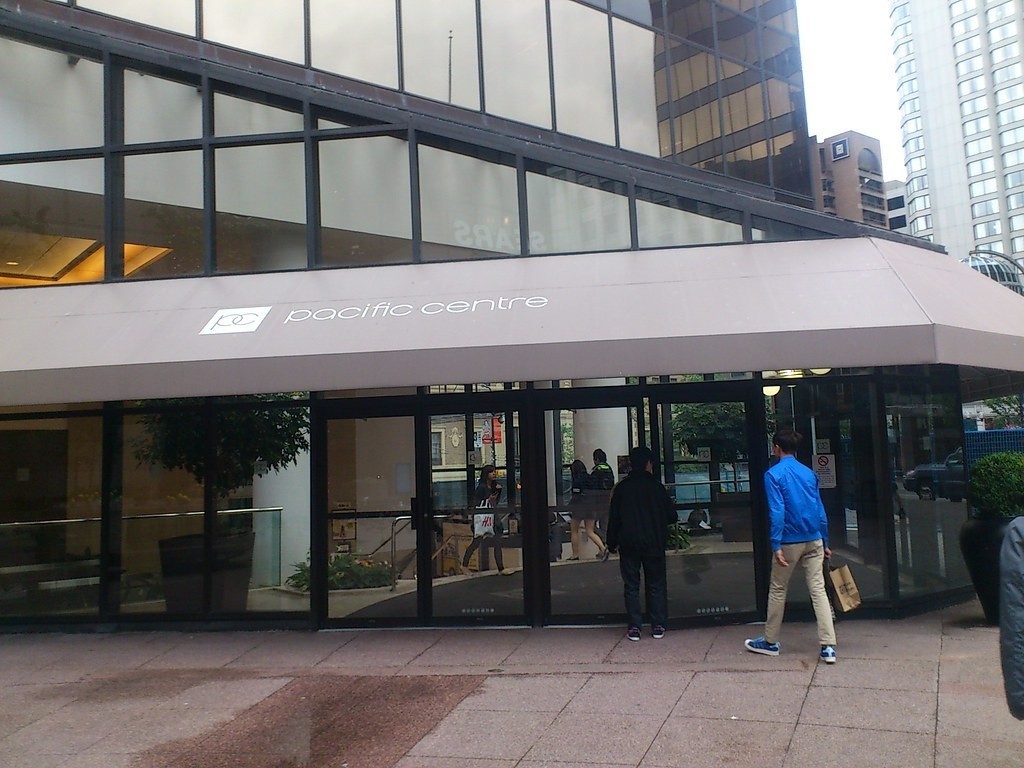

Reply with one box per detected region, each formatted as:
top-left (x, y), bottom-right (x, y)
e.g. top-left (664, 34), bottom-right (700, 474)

top-left (958, 452), bottom-right (1024, 627)
top-left (672, 396), bottom-right (781, 543)
top-left (137, 391), bottom-right (312, 618)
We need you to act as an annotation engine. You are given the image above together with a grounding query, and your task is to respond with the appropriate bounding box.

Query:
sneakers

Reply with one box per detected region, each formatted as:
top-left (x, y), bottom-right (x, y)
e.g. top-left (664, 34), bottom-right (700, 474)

top-left (819, 645), bottom-right (836, 663)
top-left (744, 636), bottom-right (780, 656)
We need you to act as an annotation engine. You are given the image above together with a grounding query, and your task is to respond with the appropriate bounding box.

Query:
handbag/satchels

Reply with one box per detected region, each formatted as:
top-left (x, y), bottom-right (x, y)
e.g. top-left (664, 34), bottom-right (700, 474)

top-left (823, 557), bottom-right (861, 612)
top-left (473, 499), bottom-right (495, 539)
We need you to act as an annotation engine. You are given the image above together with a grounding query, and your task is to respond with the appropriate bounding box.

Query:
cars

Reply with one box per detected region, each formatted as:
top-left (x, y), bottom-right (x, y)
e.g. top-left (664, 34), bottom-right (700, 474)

top-left (901, 462), bottom-right (966, 502)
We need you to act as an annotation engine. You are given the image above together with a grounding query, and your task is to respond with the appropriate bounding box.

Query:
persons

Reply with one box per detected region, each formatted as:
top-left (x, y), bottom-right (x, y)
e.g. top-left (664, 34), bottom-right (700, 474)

top-left (568, 449), bottom-right (614, 563)
top-left (460, 465), bottom-right (515, 576)
top-left (745, 430), bottom-right (837, 663)
top-left (1000, 517), bottom-right (1024, 721)
top-left (607, 447), bottom-right (677, 641)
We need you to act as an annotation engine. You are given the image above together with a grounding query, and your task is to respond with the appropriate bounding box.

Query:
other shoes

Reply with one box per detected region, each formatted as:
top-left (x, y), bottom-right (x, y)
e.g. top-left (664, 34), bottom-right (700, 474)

top-left (602, 548), bottom-right (609, 561)
top-left (566, 556), bottom-right (579, 562)
top-left (498, 569), bottom-right (514, 576)
top-left (460, 564), bottom-right (473, 576)
top-left (627, 628), bottom-right (641, 641)
top-left (652, 626), bottom-right (665, 638)
